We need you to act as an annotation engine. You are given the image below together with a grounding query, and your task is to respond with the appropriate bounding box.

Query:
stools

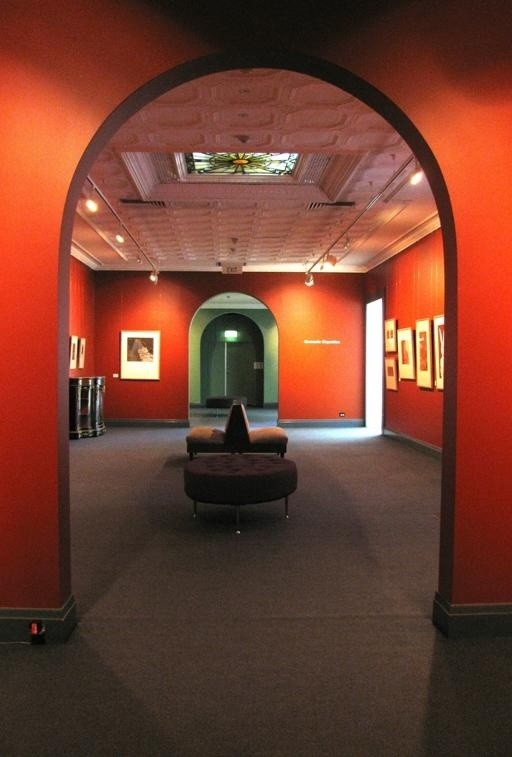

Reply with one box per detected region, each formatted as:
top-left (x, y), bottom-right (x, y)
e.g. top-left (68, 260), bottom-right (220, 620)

top-left (182, 454), bottom-right (297, 535)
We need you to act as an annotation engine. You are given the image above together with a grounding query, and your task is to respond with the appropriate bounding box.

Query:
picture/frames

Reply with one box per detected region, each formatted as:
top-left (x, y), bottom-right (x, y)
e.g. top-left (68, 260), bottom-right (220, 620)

top-left (119, 329), bottom-right (161, 382)
top-left (69, 335), bottom-right (87, 370)
top-left (384, 314), bottom-right (445, 392)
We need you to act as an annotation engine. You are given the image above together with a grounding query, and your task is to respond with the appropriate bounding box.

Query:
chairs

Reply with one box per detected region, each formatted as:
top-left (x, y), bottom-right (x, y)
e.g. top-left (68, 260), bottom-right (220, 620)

top-left (186, 399), bottom-right (288, 462)
